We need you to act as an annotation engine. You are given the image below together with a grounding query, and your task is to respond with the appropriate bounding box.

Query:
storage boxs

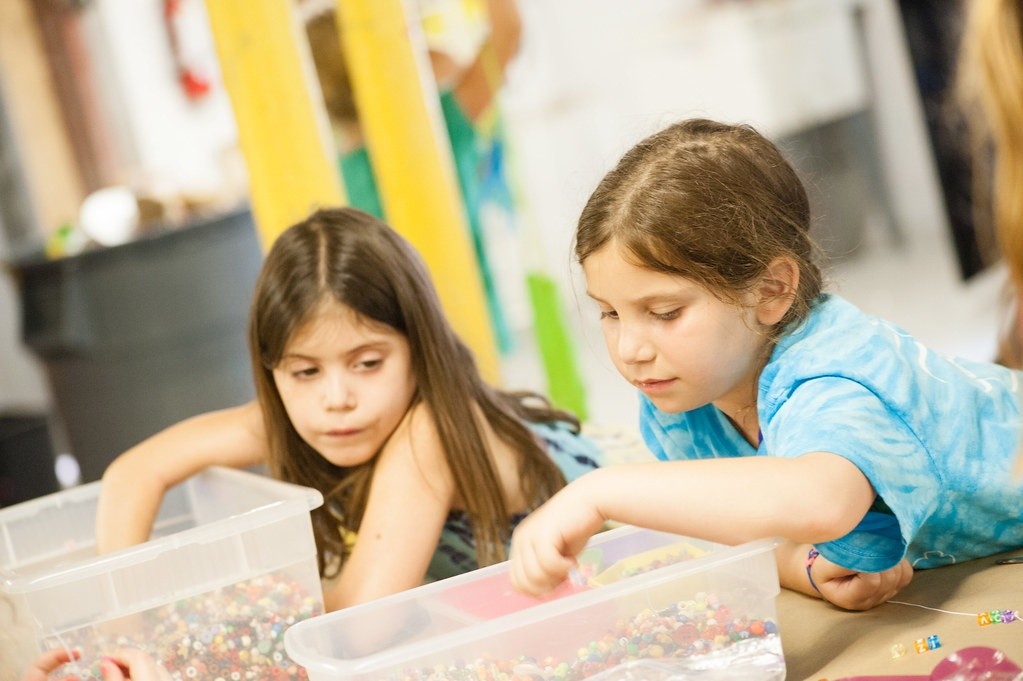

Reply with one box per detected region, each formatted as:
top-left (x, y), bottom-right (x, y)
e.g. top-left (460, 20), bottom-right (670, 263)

top-left (0, 464), bottom-right (324, 681)
top-left (284, 522), bottom-right (788, 681)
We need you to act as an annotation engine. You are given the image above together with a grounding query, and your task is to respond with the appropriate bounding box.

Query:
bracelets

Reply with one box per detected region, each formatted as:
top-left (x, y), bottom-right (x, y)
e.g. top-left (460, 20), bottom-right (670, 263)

top-left (806, 548), bottom-right (820, 593)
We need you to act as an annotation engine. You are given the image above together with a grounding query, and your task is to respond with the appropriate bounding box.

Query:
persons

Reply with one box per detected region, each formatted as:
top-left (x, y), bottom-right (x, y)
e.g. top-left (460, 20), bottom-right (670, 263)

top-left (96, 210), bottom-right (608, 614)
top-left (509, 117), bottom-right (1022, 612)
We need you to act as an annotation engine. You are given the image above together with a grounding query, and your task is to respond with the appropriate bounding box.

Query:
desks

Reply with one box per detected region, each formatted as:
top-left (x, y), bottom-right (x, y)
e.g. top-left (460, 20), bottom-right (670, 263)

top-left (775, 550), bottom-right (1022, 680)
